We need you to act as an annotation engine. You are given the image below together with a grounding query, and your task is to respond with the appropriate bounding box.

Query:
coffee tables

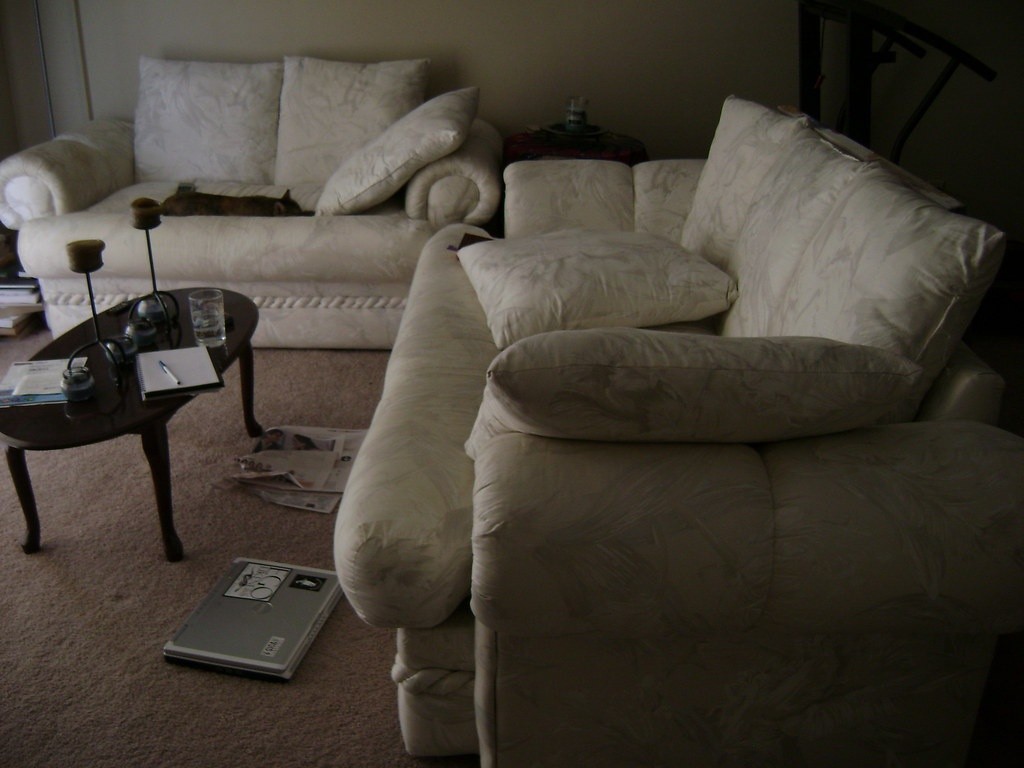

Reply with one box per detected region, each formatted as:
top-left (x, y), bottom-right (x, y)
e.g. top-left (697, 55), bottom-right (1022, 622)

top-left (0, 283), bottom-right (262, 561)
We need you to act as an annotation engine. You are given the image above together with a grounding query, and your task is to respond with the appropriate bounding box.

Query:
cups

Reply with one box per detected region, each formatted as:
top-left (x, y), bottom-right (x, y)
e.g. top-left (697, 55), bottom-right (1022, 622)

top-left (188, 289), bottom-right (226, 348)
top-left (565, 96), bottom-right (589, 133)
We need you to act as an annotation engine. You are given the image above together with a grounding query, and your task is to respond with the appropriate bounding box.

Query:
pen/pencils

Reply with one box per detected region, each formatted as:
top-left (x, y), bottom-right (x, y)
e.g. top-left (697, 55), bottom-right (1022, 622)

top-left (159, 360), bottom-right (182, 386)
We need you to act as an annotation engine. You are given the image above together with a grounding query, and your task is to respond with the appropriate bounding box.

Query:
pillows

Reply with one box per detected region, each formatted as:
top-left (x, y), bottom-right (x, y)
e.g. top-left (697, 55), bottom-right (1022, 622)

top-left (134, 55), bottom-right (284, 188)
top-left (272, 54), bottom-right (433, 189)
top-left (313, 84), bottom-right (483, 216)
top-left (466, 322), bottom-right (928, 471)
top-left (678, 94), bottom-right (1007, 428)
top-left (453, 220), bottom-right (739, 353)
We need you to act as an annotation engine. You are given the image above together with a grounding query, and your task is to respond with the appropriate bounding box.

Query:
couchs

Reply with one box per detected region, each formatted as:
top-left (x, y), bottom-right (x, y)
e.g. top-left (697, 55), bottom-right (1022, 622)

top-left (333, 156), bottom-right (1023, 768)
top-left (0, 112), bottom-right (503, 350)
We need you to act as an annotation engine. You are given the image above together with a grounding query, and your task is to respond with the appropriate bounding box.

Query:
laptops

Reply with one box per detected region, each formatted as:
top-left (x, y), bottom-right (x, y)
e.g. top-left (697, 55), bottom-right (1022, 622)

top-left (163, 556), bottom-right (343, 680)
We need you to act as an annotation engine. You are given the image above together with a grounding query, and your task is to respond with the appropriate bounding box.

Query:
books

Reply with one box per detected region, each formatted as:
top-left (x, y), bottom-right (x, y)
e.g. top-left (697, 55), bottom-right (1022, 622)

top-left (136, 345), bottom-right (226, 401)
top-left (0, 314), bottom-right (28, 336)
top-left (0, 276), bottom-right (41, 305)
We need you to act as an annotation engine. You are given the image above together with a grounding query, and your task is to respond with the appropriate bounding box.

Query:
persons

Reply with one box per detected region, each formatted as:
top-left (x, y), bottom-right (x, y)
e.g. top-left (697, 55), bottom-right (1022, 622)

top-left (291, 434), bottom-right (323, 451)
top-left (253, 429), bottom-right (284, 453)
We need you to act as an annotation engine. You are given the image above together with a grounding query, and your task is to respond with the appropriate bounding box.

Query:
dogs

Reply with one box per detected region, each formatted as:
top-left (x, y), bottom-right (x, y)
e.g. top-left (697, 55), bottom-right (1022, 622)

top-left (160, 181), bottom-right (316, 216)
